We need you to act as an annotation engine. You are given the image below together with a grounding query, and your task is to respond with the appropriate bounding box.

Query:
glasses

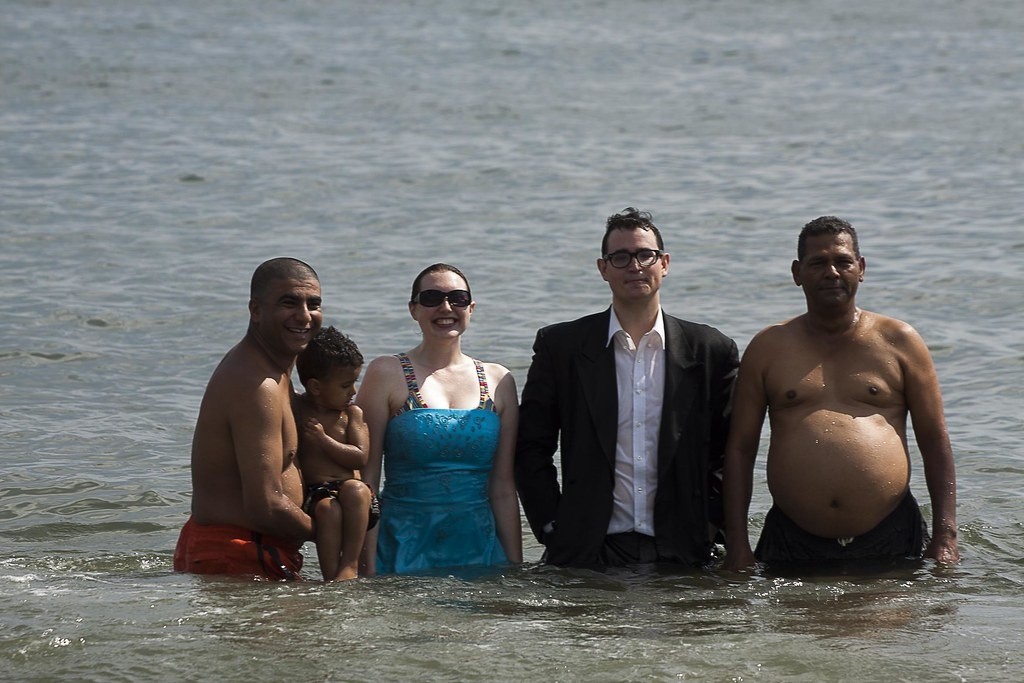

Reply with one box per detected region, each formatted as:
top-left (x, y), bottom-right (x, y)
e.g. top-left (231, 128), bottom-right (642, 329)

top-left (603, 248), bottom-right (666, 269)
top-left (411, 288), bottom-right (471, 307)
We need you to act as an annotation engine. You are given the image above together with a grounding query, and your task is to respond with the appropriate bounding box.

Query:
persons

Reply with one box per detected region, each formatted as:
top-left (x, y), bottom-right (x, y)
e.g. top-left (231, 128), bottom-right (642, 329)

top-left (356, 264), bottom-right (522, 577)
top-left (173, 258), bottom-right (380, 584)
top-left (514, 206), bottom-right (738, 565)
top-left (725, 216), bottom-right (958, 579)
